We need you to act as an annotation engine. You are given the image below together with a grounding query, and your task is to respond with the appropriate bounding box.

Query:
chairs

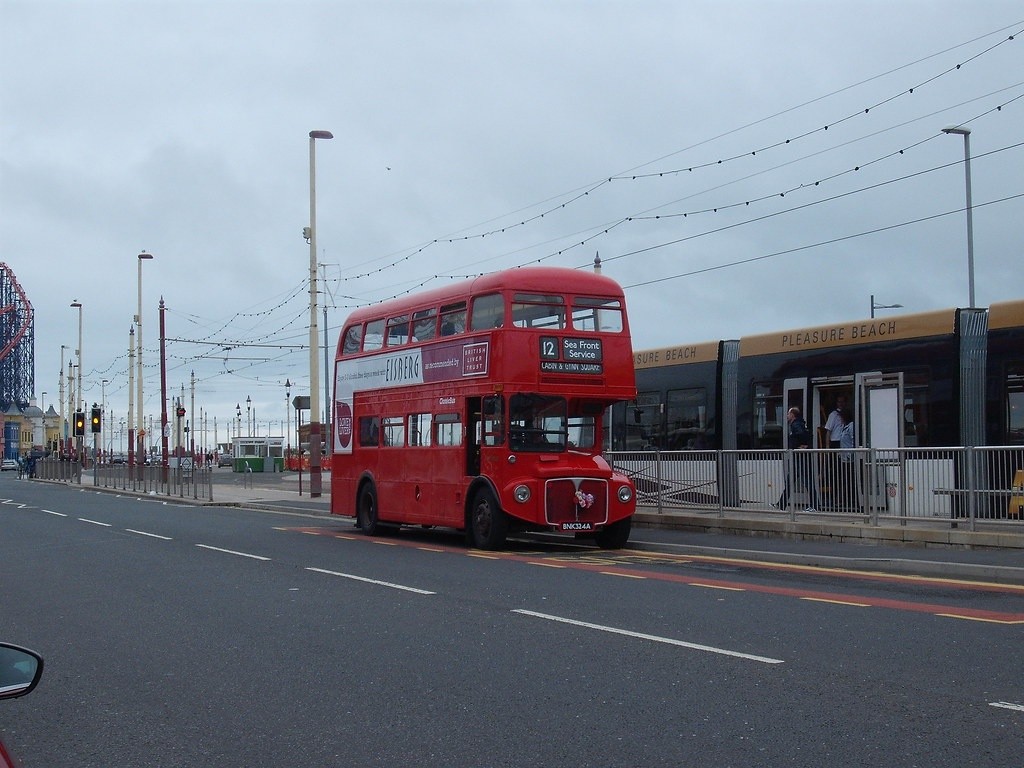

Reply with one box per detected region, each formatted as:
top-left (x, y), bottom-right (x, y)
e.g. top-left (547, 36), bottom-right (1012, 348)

top-left (762, 421), bottom-right (783, 439)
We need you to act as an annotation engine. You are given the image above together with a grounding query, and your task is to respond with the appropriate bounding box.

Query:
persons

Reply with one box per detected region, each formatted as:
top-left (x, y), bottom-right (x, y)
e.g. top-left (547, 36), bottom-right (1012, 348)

top-left (769, 406), bottom-right (822, 512)
top-left (206, 451), bottom-right (214, 467)
top-left (824, 395), bottom-right (855, 507)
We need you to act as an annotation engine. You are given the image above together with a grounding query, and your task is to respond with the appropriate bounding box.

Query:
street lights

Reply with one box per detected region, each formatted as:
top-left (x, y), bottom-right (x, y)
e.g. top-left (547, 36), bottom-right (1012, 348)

top-left (305, 130), bottom-right (334, 499)
top-left (283, 379), bottom-right (292, 460)
top-left (235, 402), bottom-right (242, 439)
top-left (871, 294), bottom-right (903, 318)
top-left (245, 394), bottom-right (252, 436)
top-left (70, 299), bottom-right (81, 485)
top-left (941, 124), bottom-right (975, 308)
top-left (102, 379), bottom-right (108, 464)
top-left (134, 249), bottom-right (153, 483)
top-left (41, 392), bottom-right (47, 460)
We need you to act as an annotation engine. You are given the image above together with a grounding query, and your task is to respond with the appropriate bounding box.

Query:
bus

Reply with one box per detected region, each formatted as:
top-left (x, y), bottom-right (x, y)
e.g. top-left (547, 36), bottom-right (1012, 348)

top-left (575, 299), bottom-right (1024, 519)
top-left (330, 267), bottom-right (638, 551)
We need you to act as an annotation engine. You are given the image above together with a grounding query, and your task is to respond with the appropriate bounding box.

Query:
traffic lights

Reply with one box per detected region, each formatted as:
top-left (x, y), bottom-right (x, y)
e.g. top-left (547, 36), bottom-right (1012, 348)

top-left (177, 407), bottom-right (187, 418)
top-left (75, 412), bottom-right (85, 437)
top-left (92, 407), bottom-right (101, 433)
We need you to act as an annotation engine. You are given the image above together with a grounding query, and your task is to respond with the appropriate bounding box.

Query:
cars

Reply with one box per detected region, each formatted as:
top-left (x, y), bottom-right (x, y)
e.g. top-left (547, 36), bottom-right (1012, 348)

top-left (113, 453), bottom-right (163, 466)
top-left (217, 454), bottom-right (233, 468)
top-left (1, 459), bottom-right (19, 472)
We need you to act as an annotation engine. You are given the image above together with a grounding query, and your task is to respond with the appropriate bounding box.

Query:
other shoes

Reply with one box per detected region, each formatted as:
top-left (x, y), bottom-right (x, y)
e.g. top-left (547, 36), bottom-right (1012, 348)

top-left (804, 506), bottom-right (821, 512)
top-left (769, 503), bottom-right (783, 511)
